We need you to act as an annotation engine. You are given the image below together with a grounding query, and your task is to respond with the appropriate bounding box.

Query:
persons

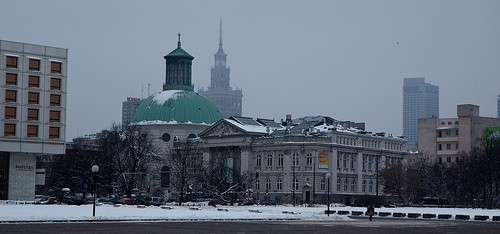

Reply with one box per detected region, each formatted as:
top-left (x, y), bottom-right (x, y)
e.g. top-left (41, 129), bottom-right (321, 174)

top-left (365, 204), bottom-right (374, 221)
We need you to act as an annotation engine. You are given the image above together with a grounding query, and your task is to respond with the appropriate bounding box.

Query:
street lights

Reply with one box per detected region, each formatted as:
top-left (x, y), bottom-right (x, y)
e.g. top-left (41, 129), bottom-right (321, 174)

top-left (92, 165), bottom-right (99, 217)
top-left (326, 173), bottom-right (331, 217)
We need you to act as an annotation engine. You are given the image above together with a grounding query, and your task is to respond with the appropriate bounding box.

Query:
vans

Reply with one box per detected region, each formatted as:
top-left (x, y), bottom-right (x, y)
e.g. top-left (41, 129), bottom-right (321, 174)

top-left (143, 196), bottom-right (164, 206)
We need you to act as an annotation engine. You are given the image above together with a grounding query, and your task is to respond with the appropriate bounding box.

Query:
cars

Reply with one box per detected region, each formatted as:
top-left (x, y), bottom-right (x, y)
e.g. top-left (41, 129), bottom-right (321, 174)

top-left (35, 194), bottom-right (94, 205)
top-left (87, 194), bottom-right (145, 205)
